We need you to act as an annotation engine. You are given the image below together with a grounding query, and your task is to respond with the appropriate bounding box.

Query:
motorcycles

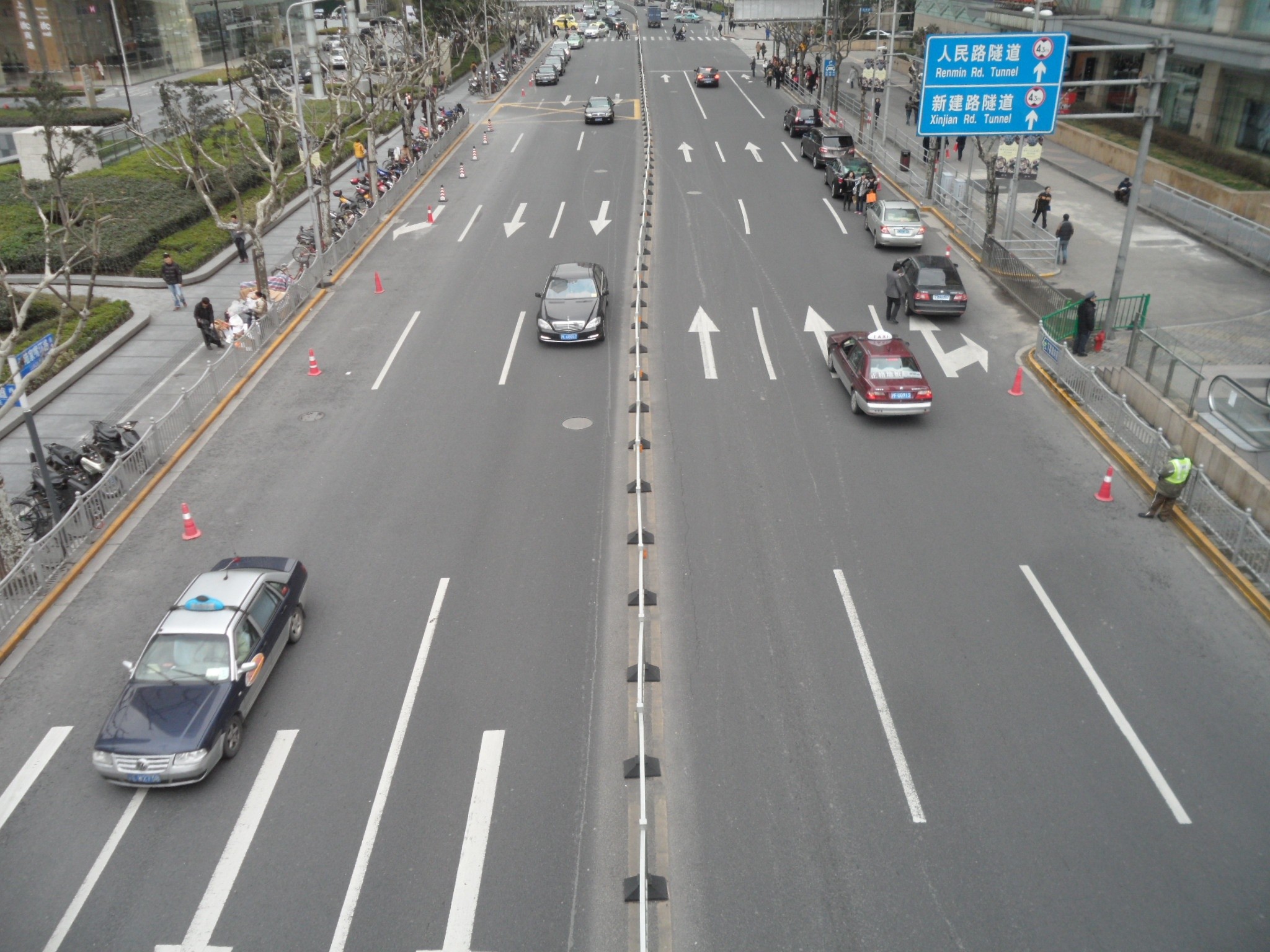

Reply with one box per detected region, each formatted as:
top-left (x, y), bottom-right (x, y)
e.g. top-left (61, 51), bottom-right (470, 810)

top-left (89, 418), bottom-right (150, 473)
top-left (27, 451), bottom-right (105, 522)
top-left (674, 30), bottom-right (687, 41)
top-left (44, 433), bottom-right (125, 499)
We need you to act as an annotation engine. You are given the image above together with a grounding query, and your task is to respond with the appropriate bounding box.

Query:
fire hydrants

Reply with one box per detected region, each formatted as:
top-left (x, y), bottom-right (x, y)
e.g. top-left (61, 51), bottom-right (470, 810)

top-left (1093, 329), bottom-right (1107, 352)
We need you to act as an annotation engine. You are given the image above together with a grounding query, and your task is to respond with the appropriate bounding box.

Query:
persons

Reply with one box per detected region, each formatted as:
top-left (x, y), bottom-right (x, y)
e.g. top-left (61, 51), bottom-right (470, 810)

top-left (885, 261), bottom-right (904, 326)
top-left (243, 289), bottom-right (269, 331)
top-left (192, 296), bottom-right (226, 350)
top-left (467, 7), bottom-right (630, 78)
top-left (668, 0), bottom-right (969, 162)
top-left (162, 252), bottom-right (186, 309)
top-left (1032, 186), bottom-right (1052, 231)
top-left (1123, 183), bottom-right (1133, 205)
top-left (229, 213), bottom-right (250, 264)
top-left (1073, 290), bottom-right (1097, 356)
top-left (1115, 177), bottom-right (1131, 202)
top-left (840, 170), bottom-right (881, 215)
top-left (1055, 213), bottom-right (1075, 265)
top-left (1139, 445), bottom-right (1192, 522)
top-left (351, 68), bottom-right (448, 176)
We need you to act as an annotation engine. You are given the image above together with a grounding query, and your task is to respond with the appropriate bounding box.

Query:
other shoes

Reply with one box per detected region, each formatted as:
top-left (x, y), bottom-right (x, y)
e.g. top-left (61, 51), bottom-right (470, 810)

top-left (1057, 259), bottom-right (1066, 266)
top-left (181, 301), bottom-right (187, 307)
top-left (1072, 350), bottom-right (1087, 356)
top-left (217, 344), bottom-right (225, 348)
top-left (887, 318), bottom-right (899, 324)
top-left (208, 346), bottom-right (212, 350)
top-left (1157, 513), bottom-right (1166, 522)
top-left (1031, 224), bottom-right (1035, 227)
top-left (1138, 512), bottom-right (1155, 518)
top-left (173, 308), bottom-right (181, 311)
top-left (844, 208), bottom-right (867, 216)
top-left (239, 258), bottom-right (248, 263)
top-left (1042, 228), bottom-right (1048, 231)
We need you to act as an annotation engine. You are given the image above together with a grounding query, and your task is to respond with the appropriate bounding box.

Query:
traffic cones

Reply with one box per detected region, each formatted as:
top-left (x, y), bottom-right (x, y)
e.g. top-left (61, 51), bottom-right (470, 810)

top-left (487, 119), bottom-right (494, 133)
top-left (177, 501), bottom-right (202, 540)
top-left (483, 130), bottom-right (488, 145)
top-left (1007, 366), bottom-right (1025, 395)
top-left (1093, 466), bottom-right (1116, 501)
top-left (471, 145), bottom-right (478, 161)
top-left (306, 347), bottom-right (323, 377)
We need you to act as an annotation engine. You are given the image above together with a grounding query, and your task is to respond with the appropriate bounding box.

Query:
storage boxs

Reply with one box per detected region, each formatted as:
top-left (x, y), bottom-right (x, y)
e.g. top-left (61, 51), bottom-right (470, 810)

top-left (357, 177), bottom-right (366, 183)
top-left (421, 117), bottom-right (427, 123)
top-left (350, 179), bottom-right (360, 184)
top-left (388, 148), bottom-right (395, 156)
top-left (333, 190), bottom-right (343, 197)
top-left (364, 172), bottom-right (370, 179)
top-left (439, 106), bottom-right (445, 111)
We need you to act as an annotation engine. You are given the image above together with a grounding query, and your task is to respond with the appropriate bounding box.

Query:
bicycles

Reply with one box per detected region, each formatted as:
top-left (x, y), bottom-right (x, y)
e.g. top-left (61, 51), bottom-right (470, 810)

top-left (467, 35), bottom-right (541, 97)
top-left (213, 99), bottom-right (466, 352)
top-left (7, 460), bottom-right (95, 568)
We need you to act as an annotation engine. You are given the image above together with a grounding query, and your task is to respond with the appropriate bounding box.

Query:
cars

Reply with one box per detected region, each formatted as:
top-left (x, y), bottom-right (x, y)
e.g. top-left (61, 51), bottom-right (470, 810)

top-left (635, 0), bottom-right (704, 28)
top-left (535, 0), bottom-right (625, 85)
top-left (583, 96), bottom-right (616, 124)
top-left (93, 541), bottom-right (307, 787)
top-left (825, 156), bottom-right (878, 200)
top-left (827, 330), bottom-right (932, 419)
top-left (864, 198), bottom-right (925, 248)
top-left (800, 124), bottom-right (857, 165)
top-left (893, 251), bottom-right (969, 316)
top-left (247, 4), bottom-right (426, 89)
top-left (536, 261), bottom-right (609, 346)
top-left (783, 103), bottom-right (823, 137)
top-left (693, 65), bottom-right (720, 87)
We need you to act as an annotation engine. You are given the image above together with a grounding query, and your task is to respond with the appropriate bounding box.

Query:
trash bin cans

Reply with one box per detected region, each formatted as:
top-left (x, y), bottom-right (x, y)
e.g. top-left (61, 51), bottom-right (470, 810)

top-left (900, 149), bottom-right (911, 171)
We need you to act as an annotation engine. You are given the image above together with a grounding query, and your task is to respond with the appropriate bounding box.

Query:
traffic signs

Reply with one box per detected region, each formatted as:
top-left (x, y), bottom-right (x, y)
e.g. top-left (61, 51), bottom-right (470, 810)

top-left (915, 31), bottom-right (1069, 137)
